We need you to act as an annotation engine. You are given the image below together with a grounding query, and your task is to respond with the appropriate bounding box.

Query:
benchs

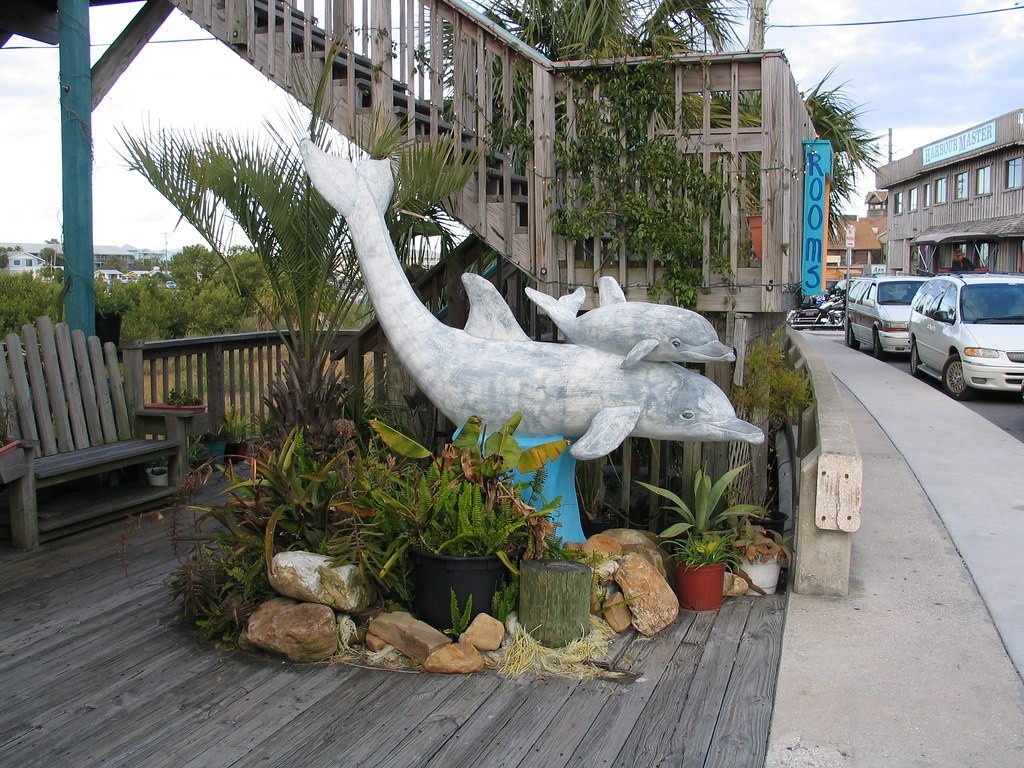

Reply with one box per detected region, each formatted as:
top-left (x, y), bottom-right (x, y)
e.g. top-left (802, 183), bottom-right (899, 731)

top-left (0, 315), bottom-right (194, 551)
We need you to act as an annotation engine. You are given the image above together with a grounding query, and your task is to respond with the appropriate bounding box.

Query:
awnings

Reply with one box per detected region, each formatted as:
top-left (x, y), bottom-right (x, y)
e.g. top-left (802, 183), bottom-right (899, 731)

top-left (907, 213), bottom-right (1024, 277)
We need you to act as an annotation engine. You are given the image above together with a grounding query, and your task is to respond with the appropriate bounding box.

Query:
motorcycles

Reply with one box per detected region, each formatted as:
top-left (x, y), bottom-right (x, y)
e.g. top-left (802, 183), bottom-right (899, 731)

top-left (788, 282), bottom-right (847, 335)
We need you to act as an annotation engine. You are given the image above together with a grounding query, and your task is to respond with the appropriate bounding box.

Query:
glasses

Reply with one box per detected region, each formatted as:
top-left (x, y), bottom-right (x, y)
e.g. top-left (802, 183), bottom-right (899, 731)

top-left (957, 253), bottom-right (962, 257)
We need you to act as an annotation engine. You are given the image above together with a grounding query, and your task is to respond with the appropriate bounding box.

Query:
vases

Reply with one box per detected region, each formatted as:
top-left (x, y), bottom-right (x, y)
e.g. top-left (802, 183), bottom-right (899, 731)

top-left (95, 313), bottom-right (122, 365)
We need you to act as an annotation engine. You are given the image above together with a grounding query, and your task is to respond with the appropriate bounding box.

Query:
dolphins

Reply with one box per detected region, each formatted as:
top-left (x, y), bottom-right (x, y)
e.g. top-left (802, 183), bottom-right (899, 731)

top-left (299, 138), bottom-right (766, 461)
top-left (525, 276), bottom-right (736, 370)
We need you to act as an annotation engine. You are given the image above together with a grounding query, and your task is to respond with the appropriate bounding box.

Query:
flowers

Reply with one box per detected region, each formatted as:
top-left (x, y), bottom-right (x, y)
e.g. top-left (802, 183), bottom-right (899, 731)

top-left (95, 290), bottom-right (133, 320)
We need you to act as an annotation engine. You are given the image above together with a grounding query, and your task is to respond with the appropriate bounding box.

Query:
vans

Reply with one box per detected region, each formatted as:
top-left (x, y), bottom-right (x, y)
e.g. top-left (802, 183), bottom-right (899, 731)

top-left (909, 271), bottom-right (1024, 400)
top-left (845, 273), bottom-right (936, 358)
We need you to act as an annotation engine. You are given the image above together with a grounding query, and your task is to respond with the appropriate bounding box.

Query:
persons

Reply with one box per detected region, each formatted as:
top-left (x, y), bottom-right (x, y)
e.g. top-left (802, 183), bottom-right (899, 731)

top-left (952, 248), bottom-right (975, 273)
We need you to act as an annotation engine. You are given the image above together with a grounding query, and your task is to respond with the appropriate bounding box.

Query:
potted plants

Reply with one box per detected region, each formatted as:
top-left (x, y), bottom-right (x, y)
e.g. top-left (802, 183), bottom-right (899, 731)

top-left (733, 516), bottom-right (797, 597)
top-left (0, 391), bottom-right (21, 456)
top-left (144, 387), bottom-right (255, 486)
top-left (657, 530), bottom-right (743, 611)
top-left (408, 463), bottom-right (525, 643)
top-left (749, 447), bottom-right (791, 541)
